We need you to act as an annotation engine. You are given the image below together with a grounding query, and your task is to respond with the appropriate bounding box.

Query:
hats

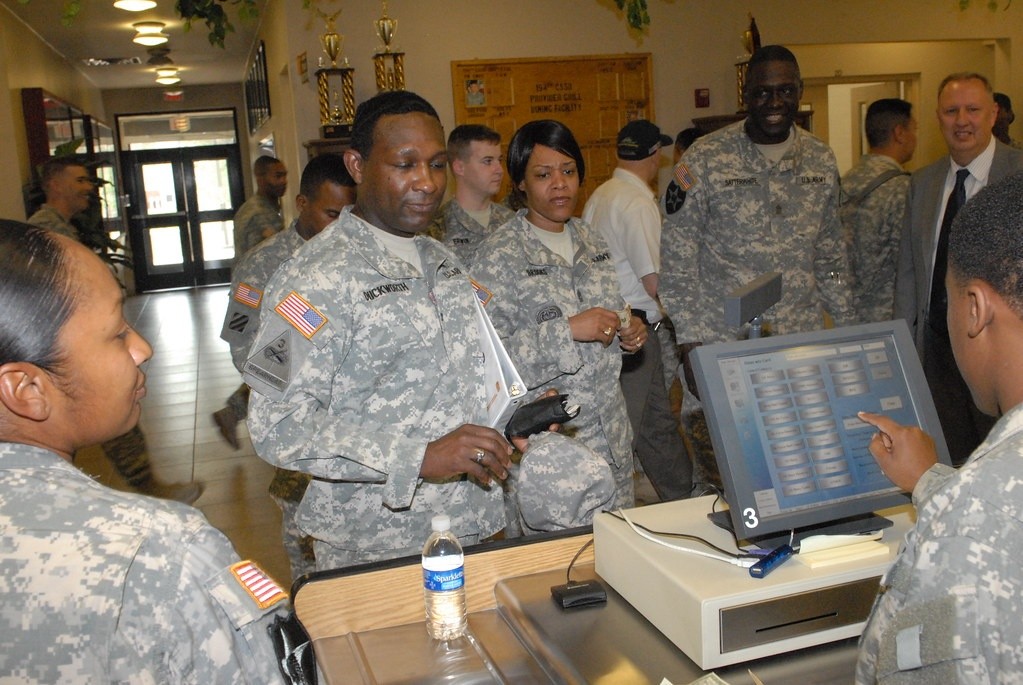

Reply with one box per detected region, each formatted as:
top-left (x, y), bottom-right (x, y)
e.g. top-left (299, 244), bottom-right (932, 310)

top-left (514, 430), bottom-right (618, 536)
top-left (615, 118), bottom-right (675, 160)
top-left (992, 91), bottom-right (1016, 125)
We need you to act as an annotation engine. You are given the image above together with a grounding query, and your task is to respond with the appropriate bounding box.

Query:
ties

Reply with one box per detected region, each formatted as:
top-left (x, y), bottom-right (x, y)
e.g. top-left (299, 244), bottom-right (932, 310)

top-left (930, 169), bottom-right (971, 338)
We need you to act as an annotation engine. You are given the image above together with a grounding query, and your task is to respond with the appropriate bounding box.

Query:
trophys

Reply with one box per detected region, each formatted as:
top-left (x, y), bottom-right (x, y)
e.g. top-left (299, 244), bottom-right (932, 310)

top-left (314, 7), bottom-right (357, 138)
top-left (369, 0), bottom-right (406, 93)
top-left (734, 18), bottom-right (759, 115)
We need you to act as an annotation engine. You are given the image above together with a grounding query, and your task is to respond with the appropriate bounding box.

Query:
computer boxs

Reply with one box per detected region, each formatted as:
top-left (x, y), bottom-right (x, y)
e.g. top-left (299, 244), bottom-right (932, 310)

top-left (592, 492), bottom-right (920, 670)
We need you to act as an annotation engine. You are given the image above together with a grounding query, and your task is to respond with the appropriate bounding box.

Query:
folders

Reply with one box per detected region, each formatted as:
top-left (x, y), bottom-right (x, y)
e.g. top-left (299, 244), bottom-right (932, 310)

top-left (472, 291), bottom-right (527, 427)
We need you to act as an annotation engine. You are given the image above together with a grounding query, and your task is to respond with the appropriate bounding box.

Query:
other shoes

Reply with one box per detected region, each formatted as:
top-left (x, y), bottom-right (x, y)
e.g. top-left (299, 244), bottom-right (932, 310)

top-left (133, 476), bottom-right (202, 505)
top-left (213, 406), bottom-right (241, 452)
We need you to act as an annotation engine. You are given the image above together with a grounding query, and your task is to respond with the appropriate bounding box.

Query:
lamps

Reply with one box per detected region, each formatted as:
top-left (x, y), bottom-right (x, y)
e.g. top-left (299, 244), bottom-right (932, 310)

top-left (155, 66), bottom-right (180, 85)
top-left (133, 22), bottom-right (170, 45)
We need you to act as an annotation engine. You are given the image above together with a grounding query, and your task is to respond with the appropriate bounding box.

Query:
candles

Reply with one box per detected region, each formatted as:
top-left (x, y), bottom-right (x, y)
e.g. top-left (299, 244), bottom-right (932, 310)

top-left (332, 86), bottom-right (338, 104)
top-left (387, 66), bottom-right (394, 89)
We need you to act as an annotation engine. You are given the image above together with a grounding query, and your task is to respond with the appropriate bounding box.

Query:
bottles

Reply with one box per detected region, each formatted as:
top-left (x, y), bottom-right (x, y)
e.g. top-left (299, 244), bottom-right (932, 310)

top-left (421, 515), bottom-right (467, 639)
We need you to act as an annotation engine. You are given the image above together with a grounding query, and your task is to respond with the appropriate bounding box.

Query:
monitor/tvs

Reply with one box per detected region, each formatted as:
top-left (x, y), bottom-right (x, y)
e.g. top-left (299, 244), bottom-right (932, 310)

top-left (688, 318), bottom-right (954, 554)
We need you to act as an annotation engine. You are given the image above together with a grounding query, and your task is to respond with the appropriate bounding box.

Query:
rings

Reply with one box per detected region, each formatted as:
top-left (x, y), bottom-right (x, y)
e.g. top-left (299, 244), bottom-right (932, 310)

top-left (635, 336), bottom-right (641, 344)
top-left (635, 342), bottom-right (642, 349)
top-left (604, 326), bottom-right (612, 336)
top-left (476, 448), bottom-right (485, 464)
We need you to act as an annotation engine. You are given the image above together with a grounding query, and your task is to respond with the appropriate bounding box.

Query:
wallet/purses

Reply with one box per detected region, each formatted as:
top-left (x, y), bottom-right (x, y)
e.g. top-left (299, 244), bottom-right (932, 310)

top-left (505, 393), bottom-right (582, 439)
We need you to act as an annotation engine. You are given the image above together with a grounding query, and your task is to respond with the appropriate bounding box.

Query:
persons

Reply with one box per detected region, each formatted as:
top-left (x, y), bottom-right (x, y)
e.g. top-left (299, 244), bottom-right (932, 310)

top-left (853, 170), bottom-right (1023, 685)
top-left (242, 89), bottom-right (560, 573)
top-left (657, 46), bottom-right (852, 500)
top-left (211, 155), bottom-right (290, 452)
top-left (0, 216), bottom-right (329, 685)
top-left (469, 119), bottom-right (649, 539)
top-left (220, 152), bottom-right (358, 586)
top-left (466, 79), bottom-right (484, 106)
top-left (581, 119), bottom-right (694, 503)
top-left (416, 124), bottom-right (516, 266)
top-left (28, 156), bottom-right (202, 503)
top-left (840, 98), bottom-right (918, 330)
top-left (673, 128), bottom-right (708, 166)
top-left (991, 93), bottom-right (1023, 151)
top-left (891, 71), bottom-right (1023, 463)
